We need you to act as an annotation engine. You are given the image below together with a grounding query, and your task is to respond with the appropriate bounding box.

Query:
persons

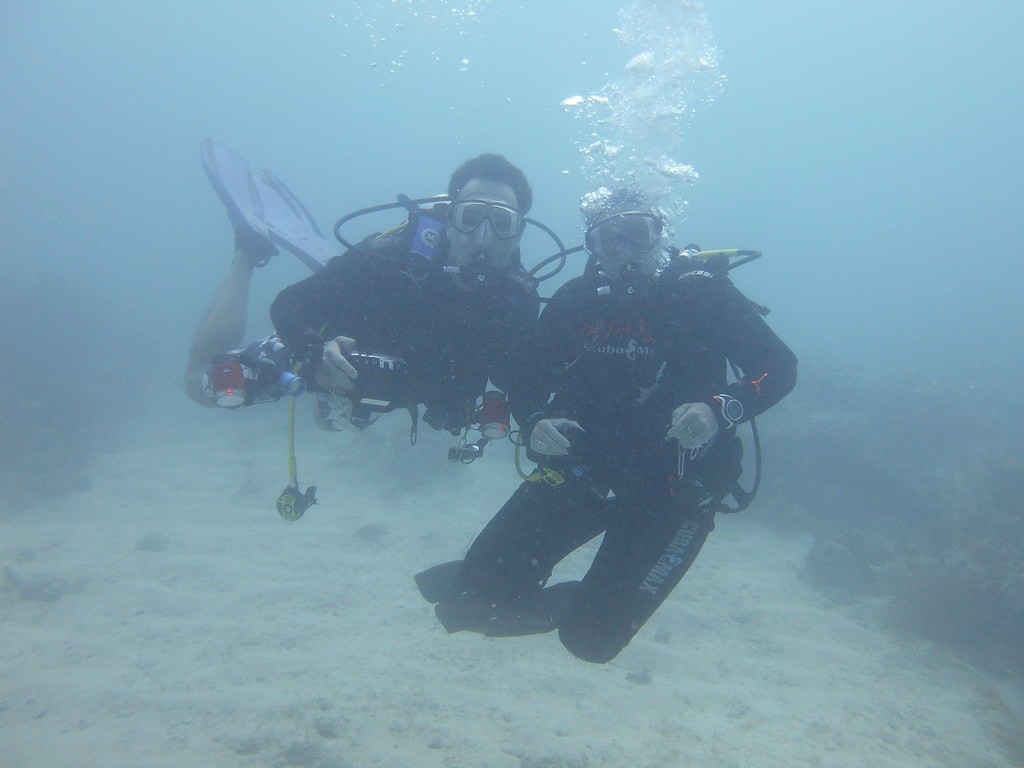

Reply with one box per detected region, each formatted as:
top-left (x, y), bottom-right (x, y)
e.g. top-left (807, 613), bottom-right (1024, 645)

top-left (414, 190), bottom-right (798, 665)
top-left (185, 134), bottom-right (540, 442)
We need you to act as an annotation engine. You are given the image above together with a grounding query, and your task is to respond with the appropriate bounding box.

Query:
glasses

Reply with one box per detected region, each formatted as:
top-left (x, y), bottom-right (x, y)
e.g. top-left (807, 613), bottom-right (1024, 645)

top-left (585, 211), bottom-right (663, 261)
top-left (450, 198), bottom-right (526, 240)
